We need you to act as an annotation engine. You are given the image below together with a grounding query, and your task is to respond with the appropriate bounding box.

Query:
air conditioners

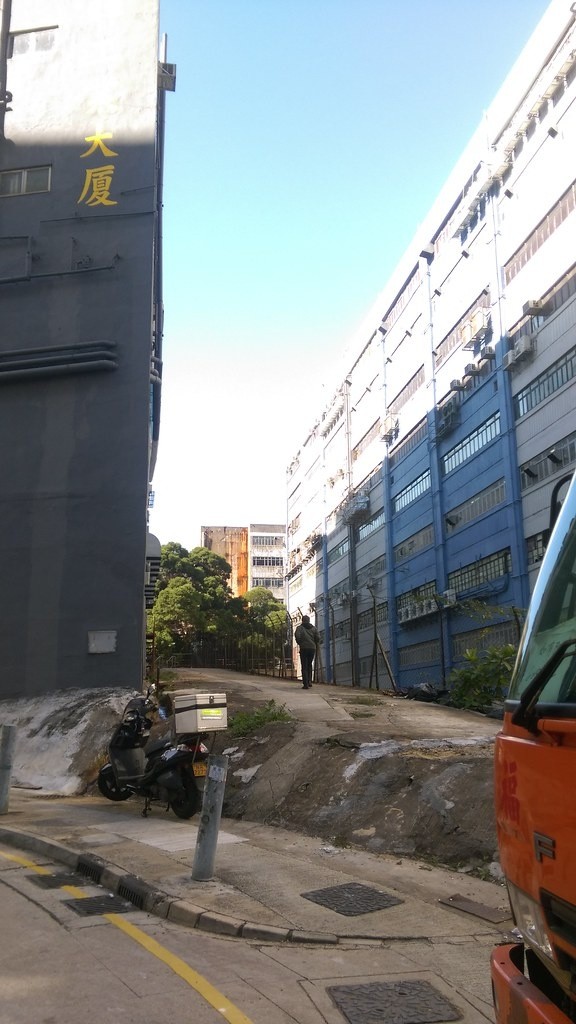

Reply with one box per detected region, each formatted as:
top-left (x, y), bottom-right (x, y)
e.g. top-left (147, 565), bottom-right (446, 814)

top-left (502, 350), bottom-right (516, 371)
top-left (442, 397), bottom-right (456, 420)
top-left (450, 380), bottom-right (460, 391)
top-left (481, 346), bottom-right (492, 359)
top-left (523, 300), bottom-right (542, 315)
top-left (397, 589), bottom-right (456, 623)
top-left (465, 364), bottom-right (475, 376)
top-left (514, 335), bottom-right (531, 360)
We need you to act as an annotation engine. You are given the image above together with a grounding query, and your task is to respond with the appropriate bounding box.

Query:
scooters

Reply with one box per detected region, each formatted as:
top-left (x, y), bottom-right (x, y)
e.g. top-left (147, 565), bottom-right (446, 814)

top-left (97, 672), bottom-right (228, 820)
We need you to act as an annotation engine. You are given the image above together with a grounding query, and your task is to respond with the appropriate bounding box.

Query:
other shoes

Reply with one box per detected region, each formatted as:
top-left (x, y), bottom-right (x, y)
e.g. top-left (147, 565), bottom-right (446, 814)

top-left (302, 683), bottom-right (308, 689)
top-left (308, 680), bottom-right (312, 687)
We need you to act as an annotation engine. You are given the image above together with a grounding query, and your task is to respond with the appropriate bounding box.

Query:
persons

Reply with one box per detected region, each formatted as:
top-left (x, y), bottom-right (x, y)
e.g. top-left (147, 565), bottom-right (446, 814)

top-left (294, 615), bottom-right (321, 690)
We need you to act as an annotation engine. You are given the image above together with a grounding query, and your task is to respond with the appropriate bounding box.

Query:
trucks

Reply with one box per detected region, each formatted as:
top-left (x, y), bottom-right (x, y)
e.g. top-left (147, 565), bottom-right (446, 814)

top-left (489, 469), bottom-right (576, 1024)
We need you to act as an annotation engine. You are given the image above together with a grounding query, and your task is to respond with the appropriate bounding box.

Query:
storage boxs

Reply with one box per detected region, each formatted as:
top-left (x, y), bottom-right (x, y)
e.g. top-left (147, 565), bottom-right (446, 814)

top-left (175, 694), bottom-right (228, 733)
